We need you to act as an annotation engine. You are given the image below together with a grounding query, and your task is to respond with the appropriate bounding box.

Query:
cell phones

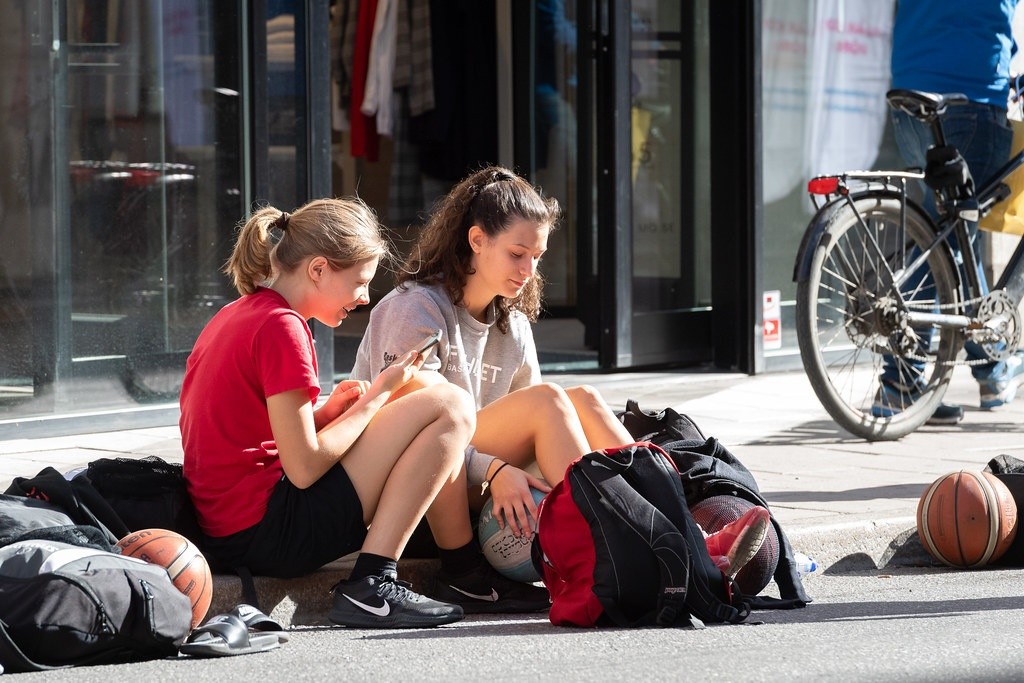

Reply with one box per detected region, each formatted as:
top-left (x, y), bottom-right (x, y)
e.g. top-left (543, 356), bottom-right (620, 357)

top-left (388, 328), bottom-right (443, 367)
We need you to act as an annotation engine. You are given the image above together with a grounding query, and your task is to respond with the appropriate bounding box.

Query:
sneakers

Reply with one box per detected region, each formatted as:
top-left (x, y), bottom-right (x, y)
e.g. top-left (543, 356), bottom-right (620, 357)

top-left (697, 506), bottom-right (771, 592)
top-left (972, 347), bottom-right (1023, 409)
top-left (871, 376), bottom-right (964, 425)
top-left (327, 569), bottom-right (465, 628)
top-left (430, 568), bottom-right (555, 612)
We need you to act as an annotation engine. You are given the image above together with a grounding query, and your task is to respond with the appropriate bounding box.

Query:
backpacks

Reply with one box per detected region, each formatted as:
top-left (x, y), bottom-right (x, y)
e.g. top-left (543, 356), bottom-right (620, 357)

top-left (0, 453), bottom-right (221, 670)
top-left (531, 398), bottom-right (813, 631)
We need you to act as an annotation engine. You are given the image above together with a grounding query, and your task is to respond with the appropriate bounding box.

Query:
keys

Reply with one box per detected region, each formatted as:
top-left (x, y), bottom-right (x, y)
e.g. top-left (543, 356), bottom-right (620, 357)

top-left (481, 462), bottom-right (509, 495)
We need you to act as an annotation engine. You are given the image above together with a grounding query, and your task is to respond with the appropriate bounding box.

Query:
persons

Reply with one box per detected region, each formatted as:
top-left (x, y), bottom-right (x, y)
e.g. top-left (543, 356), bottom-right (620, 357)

top-left (180, 199), bottom-right (553, 631)
top-left (349, 167), bottom-right (770, 591)
top-left (871, 0), bottom-right (1024, 424)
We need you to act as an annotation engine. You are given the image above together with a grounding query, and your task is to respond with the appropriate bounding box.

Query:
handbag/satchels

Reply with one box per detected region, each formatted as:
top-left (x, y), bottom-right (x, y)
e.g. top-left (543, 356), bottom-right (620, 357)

top-left (976, 75), bottom-right (1024, 237)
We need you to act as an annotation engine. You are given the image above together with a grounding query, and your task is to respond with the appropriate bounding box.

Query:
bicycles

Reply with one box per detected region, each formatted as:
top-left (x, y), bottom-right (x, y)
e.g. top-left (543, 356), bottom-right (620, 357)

top-left (69, 84), bottom-right (240, 403)
top-left (792, 71), bottom-right (1024, 442)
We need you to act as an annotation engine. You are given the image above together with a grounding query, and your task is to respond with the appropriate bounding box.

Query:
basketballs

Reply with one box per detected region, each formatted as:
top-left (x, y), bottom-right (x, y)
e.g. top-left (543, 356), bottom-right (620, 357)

top-left (476, 477), bottom-right (550, 585)
top-left (916, 468), bottom-right (1020, 572)
top-left (111, 525), bottom-right (216, 634)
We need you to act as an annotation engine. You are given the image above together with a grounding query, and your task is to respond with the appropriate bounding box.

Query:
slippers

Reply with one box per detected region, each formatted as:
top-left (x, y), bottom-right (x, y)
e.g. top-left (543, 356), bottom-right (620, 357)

top-left (180, 604), bottom-right (290, 656)
